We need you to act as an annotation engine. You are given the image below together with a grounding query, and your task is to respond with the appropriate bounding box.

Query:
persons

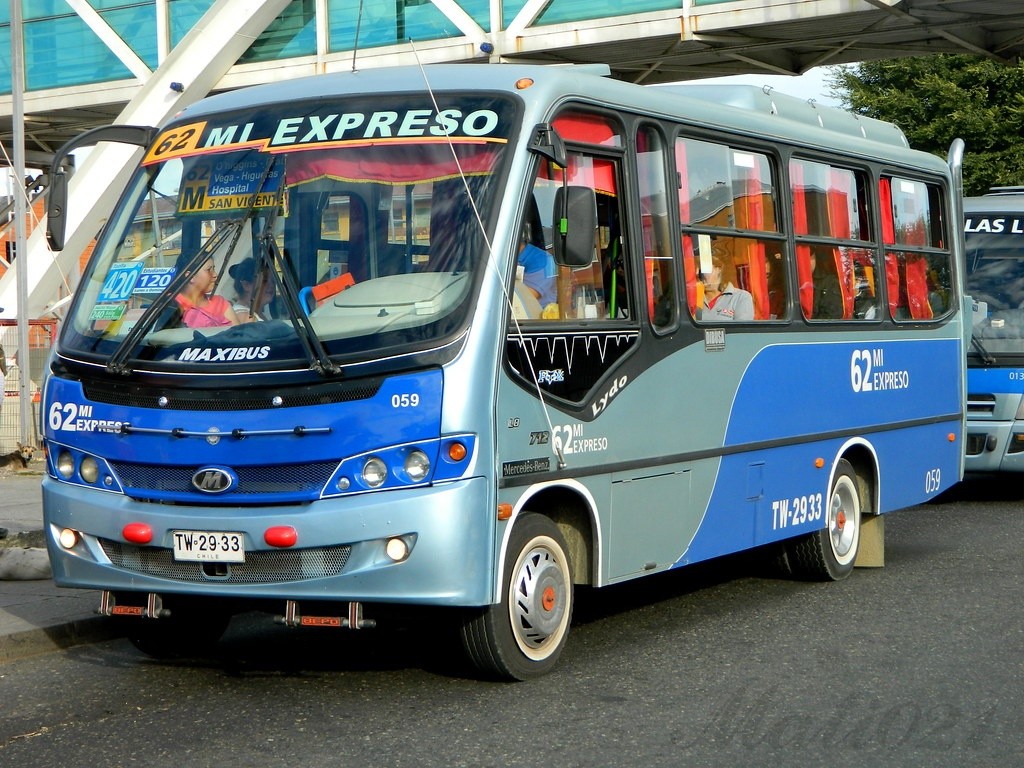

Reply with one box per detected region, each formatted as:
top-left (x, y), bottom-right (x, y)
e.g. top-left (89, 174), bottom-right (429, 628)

top-left (657, 226), bottom-right (949, 332)
top-left (229, 258), bottom-right (277, 326)
top-left (967, 262), bottom-right (1024, 310)
top-left (516, 236), bottom-right (559, 314)
top-left (160, 248), bottom-right (239, 329)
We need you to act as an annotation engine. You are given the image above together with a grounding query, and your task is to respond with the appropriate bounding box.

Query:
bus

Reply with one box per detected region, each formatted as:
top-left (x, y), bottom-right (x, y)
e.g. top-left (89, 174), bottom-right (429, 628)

top-left (958, 184), bottom-right (1024, 479)
top-left (22, 58), bottom-right (972, 683)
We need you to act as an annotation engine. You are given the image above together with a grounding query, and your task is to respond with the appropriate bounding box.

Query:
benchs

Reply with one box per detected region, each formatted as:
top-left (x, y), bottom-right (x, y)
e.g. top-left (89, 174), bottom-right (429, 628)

top-left (636, 130), bottom-right (698, 324)
top-left (894, 180), bottom-right (934, 319)
top-left (759, 158), bottom-right (814, 321)
top-left (803, 163), bottom-right (856, 319)
top-left (693, 154), bottom-right (770, 321)
top-left (843, 177), bottom-right (900, 319)
